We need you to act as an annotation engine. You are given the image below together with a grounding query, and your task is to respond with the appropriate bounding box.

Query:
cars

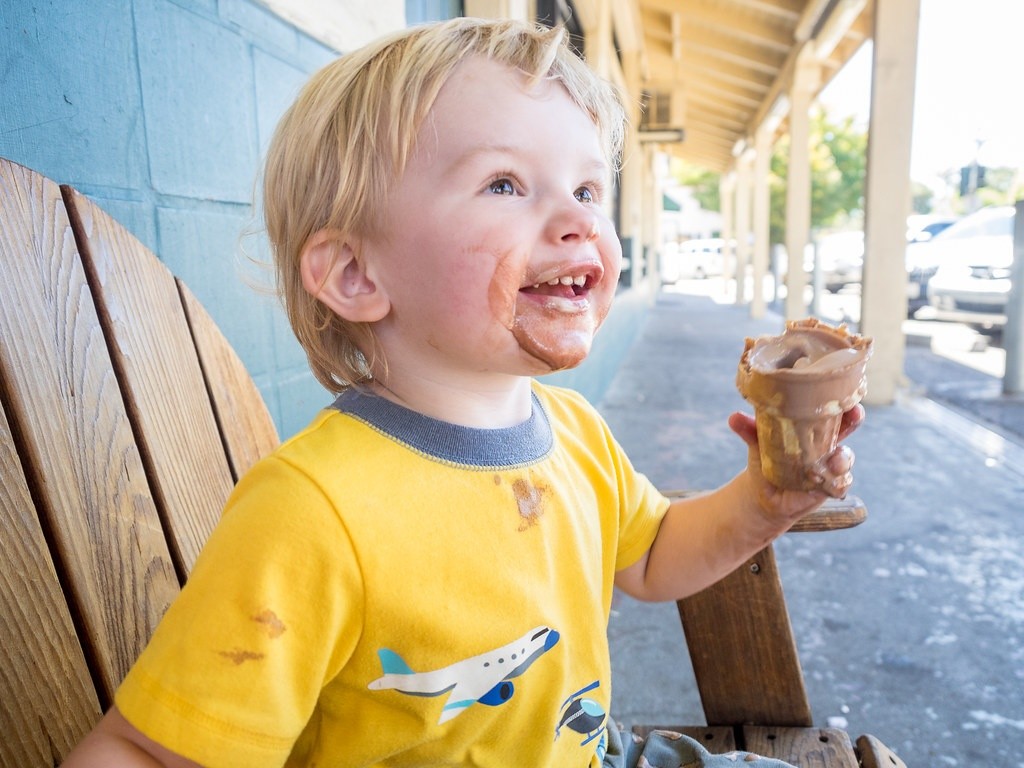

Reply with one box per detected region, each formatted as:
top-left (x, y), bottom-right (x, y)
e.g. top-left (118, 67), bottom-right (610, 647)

top-left (783, 202), bottom-right (1017, 323)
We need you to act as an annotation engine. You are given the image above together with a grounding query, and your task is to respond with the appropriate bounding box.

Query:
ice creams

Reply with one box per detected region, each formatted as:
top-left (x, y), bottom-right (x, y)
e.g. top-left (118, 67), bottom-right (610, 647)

top-left (737, 318), bottom-right (873, 490)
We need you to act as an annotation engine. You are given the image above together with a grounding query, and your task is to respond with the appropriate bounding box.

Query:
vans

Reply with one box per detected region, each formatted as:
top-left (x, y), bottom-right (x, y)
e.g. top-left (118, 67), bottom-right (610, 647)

top-left (619, 238), bottom-right (731, 287)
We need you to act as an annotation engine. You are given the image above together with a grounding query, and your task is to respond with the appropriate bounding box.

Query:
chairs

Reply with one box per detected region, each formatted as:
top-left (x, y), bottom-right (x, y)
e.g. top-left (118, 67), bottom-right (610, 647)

top-left (0, 157), bottom-right (907, 767)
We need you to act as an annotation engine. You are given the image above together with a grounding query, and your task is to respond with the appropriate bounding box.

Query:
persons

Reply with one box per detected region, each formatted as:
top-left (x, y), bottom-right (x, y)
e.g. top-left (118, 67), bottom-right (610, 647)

top-left (56, 17), bottom-right (865, 767)
